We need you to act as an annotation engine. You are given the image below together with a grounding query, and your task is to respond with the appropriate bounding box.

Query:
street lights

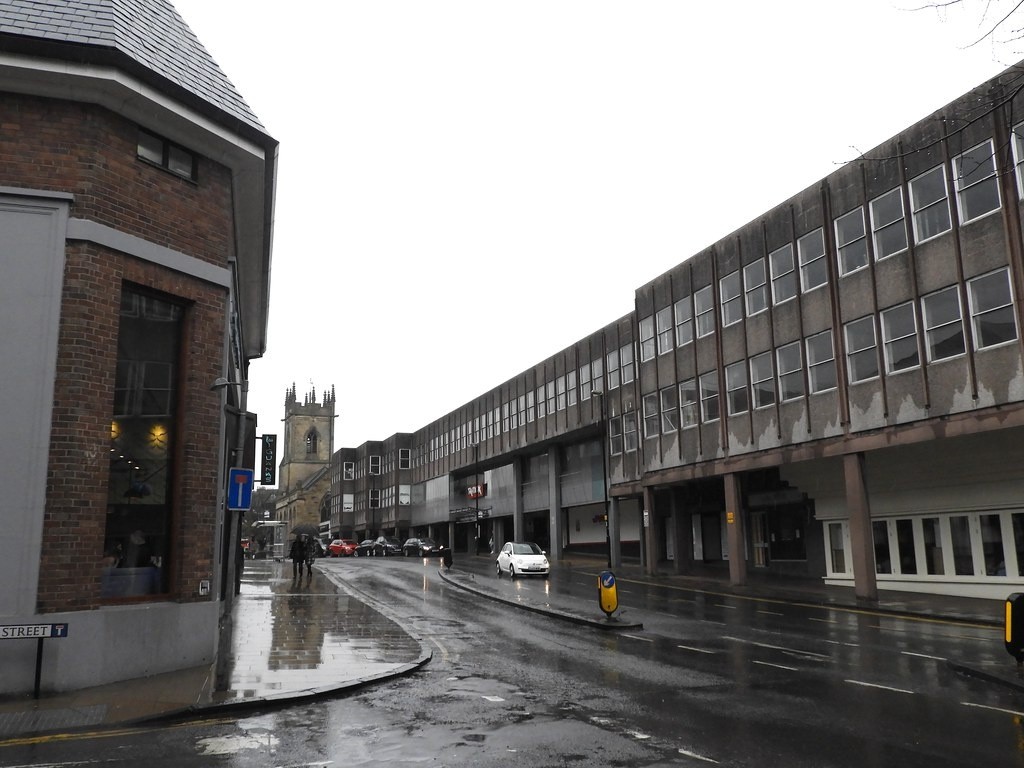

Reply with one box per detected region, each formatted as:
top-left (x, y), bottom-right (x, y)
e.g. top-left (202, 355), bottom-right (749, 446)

top-left (368, 470), bottom-right (376, 540)
top-left (469, 441), bottom-right (480, 556)
top-left (590, 388), bottom-right (612, 568)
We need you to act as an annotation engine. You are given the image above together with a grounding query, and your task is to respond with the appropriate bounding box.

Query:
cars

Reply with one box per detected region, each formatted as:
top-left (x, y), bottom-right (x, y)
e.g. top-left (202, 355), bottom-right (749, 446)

top-left (403, 537), bottom-right (444, 557)
top-left (314, 536), bottom-right (335, 557)
top-left (327, 538), bottom-right (359, 556)
top-left (371, 535), bottom-right (404, 557)
top-left (353, 539), bottom-right (376, 557)
top-left (494, 541), bottom-right (551, 578)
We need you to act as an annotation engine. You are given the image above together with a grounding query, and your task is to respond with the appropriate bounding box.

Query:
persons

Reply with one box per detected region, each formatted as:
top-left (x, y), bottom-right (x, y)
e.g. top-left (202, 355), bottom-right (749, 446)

top-left (289, 534), bottom-right (305, 578)
top-left (304, 536), bottom-right (318, 577)
top-left (474, 536), bottom-right (480, 556)
top-left (117, 530), bottom-right (150, 568)
top-left (489, 533), bottom-right (494, 554)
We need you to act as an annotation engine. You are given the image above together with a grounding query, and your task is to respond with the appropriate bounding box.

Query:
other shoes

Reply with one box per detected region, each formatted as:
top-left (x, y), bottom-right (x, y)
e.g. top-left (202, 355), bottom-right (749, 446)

top-left (292, 575), bottom-right (296, 579)
top-left (307, 571), bottom-right (309, 576)
top-left (310, 572), bottom-right (312, 576)
top-left (300, 573), bottom-right (303, 577)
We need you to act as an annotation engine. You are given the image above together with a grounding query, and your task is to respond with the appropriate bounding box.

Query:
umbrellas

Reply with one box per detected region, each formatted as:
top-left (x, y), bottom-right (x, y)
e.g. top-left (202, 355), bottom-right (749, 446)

top-left (289, 523), bottom-right (320, 537)
